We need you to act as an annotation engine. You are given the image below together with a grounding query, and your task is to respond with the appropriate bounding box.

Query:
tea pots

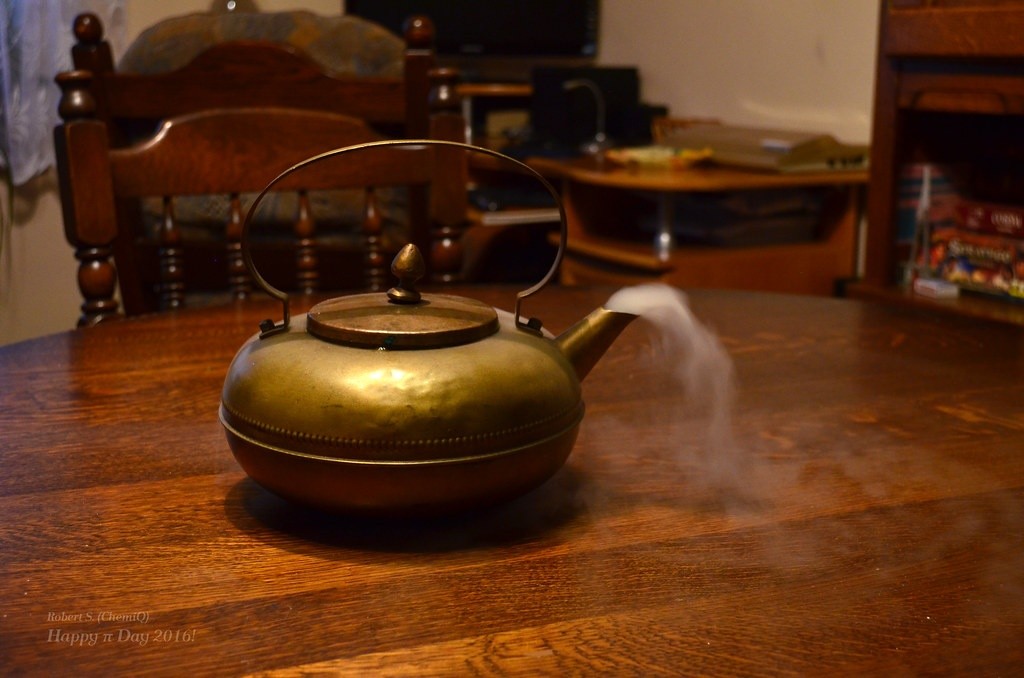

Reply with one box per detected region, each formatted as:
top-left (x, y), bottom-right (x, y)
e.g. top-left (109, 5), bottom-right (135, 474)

top-left (216, 137), bottom-right (639, 527)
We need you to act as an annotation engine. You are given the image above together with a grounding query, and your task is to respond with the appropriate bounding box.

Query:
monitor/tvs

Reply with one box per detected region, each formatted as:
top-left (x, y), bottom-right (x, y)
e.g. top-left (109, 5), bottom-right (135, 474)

top-left (344, 0), bottom-right (599, 60)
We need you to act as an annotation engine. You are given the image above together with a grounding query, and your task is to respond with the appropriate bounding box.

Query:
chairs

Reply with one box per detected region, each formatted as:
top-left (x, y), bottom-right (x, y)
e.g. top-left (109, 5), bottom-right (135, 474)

top-left (53, 69), bottom-right (468, 330)
top-left (72, 13), bottom-right (435, 313)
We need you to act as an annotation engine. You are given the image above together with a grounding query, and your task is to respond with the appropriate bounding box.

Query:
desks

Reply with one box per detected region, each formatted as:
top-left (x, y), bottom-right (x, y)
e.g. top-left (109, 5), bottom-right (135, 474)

top-left (0, 87), bottom-right (1024, 678)
